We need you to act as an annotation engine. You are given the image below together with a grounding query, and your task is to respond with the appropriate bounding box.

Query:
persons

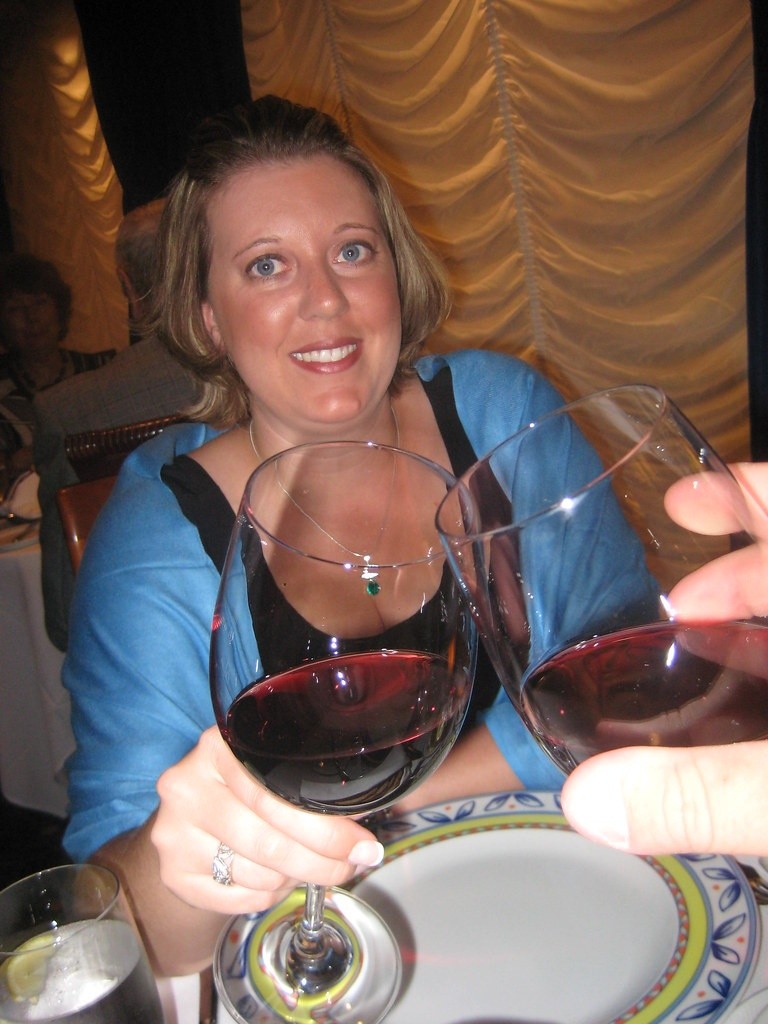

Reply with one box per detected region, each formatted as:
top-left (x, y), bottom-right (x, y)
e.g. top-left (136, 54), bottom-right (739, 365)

top-left (61, 93), bottom-right (660, 975)
top-left (0, 199), bottom-right (205, 652)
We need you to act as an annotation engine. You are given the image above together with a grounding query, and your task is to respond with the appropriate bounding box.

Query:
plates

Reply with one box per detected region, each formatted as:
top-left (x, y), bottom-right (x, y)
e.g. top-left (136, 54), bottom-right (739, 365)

top-left (213, 790), bottom-right (760, 1024)
top-left (0, 522), bottom-right (39, 551)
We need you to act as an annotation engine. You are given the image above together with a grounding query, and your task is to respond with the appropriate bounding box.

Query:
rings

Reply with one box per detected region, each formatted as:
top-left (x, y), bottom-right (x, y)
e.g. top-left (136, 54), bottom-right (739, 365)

top-left (212, 842), bottom-right (235, 885)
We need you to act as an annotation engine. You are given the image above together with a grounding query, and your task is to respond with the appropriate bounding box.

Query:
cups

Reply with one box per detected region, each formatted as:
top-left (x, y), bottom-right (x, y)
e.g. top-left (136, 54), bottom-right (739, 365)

top-left (0, 864), bottom-right (167, 1024)
top-left (435, 384), bottom-right (767, 778)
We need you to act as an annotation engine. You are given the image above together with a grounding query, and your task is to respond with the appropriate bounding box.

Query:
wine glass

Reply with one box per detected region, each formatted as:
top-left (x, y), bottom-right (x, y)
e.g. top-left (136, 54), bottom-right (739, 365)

top-left (210, 439), bottom-right (484, 1024)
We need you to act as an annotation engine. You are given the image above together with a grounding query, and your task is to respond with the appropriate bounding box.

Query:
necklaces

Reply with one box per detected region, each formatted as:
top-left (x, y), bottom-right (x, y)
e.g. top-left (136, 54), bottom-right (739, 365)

top-left (249, 408), bottom-right (400, 595)
